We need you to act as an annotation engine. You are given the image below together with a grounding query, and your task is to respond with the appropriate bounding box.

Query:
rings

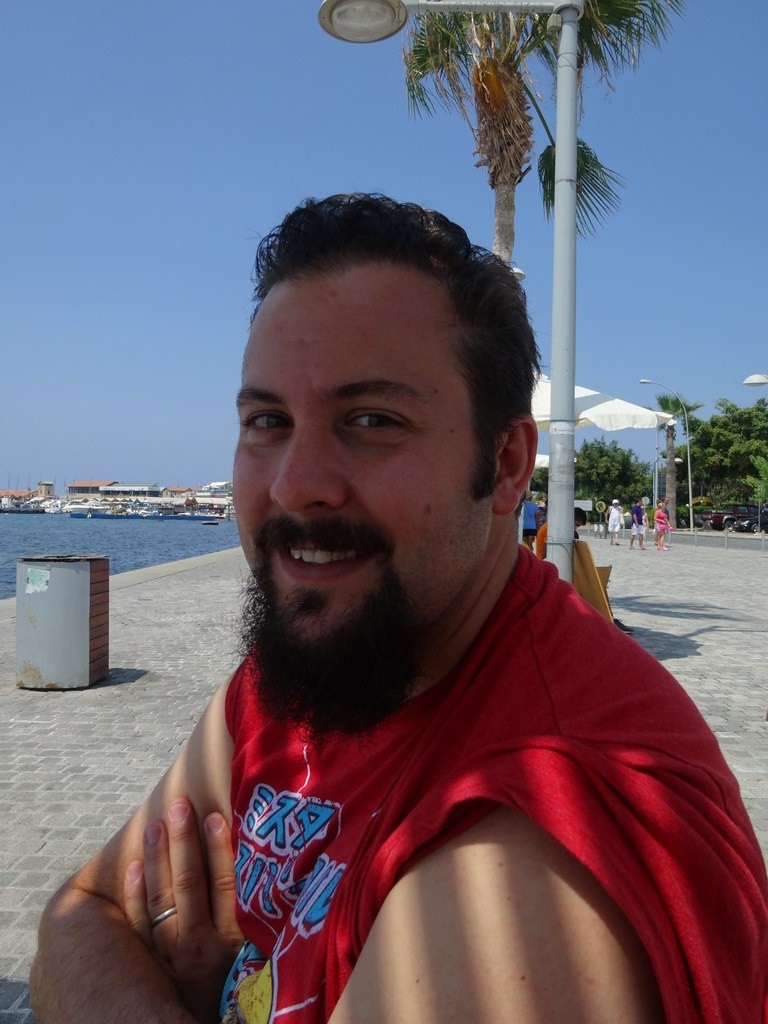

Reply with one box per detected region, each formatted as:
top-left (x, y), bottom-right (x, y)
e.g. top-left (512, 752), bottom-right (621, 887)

top-left (150, 905), bottom-right (177, 929)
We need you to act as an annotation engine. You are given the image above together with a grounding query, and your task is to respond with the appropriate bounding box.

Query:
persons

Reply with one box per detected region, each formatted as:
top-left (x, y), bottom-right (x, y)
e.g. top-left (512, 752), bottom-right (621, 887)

top-left (522, 489), bottom-right (588, 560)
top-left (605, 499), bottom-right (623, 545)
top-left (605, 591), bottom-right (634, 635)
top-left (26, 190), bottom-right (768, 1023)
top-left (628, 497), bottom-right (674, 551)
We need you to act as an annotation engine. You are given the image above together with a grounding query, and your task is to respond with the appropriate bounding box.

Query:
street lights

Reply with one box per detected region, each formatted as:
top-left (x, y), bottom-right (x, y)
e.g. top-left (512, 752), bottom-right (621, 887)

top-left (638, 379), bottom-right (695, 532)
top-left (653, 457), bottom-right (684, 513)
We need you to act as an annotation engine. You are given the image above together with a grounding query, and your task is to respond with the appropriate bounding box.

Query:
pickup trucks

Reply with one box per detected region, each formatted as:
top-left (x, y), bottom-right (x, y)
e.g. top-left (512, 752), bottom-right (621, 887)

top-left (700, 502), bottom-right (761, 531)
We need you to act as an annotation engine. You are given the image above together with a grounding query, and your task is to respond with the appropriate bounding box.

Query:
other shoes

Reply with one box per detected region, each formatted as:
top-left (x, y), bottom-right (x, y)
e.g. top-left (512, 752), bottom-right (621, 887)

top-left (615, 542), bottom-right (619, 546)
top-left (658, 546), bottom-right (669, 551)
top-left (610, 542), bottom-right (614, 545)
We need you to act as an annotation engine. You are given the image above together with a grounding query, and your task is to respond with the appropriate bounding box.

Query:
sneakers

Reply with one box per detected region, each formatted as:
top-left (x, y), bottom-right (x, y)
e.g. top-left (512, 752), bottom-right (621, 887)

top-left (613, 618), bottom-right (634, 633)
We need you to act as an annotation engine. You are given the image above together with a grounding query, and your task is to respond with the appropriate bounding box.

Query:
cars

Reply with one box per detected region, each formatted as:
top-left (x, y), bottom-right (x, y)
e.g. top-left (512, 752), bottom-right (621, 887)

top-left (733, 508), bottom-right (768, 533)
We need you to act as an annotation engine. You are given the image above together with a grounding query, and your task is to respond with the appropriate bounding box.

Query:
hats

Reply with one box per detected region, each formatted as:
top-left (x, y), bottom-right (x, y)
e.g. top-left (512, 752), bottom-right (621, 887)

top-left (612, 499), bottom-right (619, 505)
top-left (525, 492), bottom-right (532, 498)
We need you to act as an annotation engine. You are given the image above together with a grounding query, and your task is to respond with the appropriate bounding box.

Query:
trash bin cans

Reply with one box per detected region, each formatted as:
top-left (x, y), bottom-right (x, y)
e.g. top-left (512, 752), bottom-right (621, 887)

top-left (12, 553), bottom-right (111, 692)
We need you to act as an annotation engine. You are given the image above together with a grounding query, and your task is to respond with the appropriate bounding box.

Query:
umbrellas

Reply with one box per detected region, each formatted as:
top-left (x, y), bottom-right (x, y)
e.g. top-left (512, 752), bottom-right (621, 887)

top-left (528, 370), bottom-right (678, 431)
